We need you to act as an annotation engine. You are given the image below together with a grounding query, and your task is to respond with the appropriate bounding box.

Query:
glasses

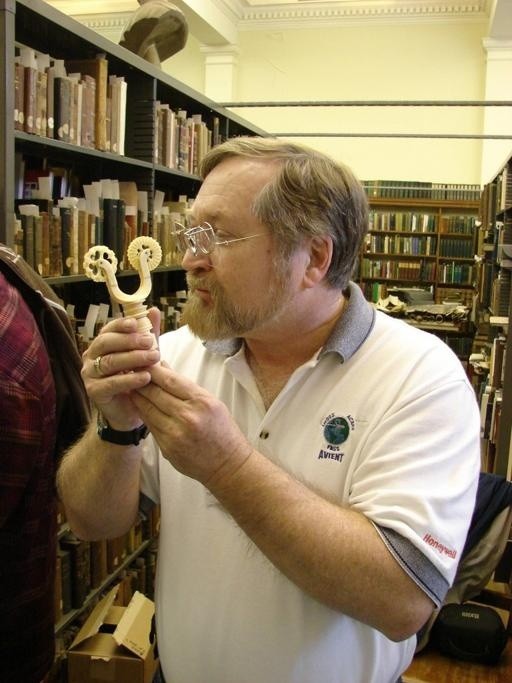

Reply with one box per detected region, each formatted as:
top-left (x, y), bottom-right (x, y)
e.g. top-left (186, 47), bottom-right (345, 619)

top-left (170, 221), bottom-right (277, 257)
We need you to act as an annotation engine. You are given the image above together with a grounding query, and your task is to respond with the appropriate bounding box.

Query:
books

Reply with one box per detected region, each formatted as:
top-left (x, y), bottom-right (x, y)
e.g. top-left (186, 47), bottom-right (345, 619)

top-left (56, 496), bottom-right (160, 680)
top-left (155, 100), bottom-right (222, 177)
top-left (14, 40), bottom-right (126, 155)
top-left (60, 291), bottom-right (189, 342)
top-left (355, 181), bottom-right (479, 330)
top-left (467, 158), bottom-right (512, 500)
top-left (14, 152), bottom-right (195, 277)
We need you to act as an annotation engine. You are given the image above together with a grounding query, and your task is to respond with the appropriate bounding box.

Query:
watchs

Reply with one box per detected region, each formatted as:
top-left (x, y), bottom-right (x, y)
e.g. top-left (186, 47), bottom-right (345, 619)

top-left (94, 408), bottom-right (151, 447)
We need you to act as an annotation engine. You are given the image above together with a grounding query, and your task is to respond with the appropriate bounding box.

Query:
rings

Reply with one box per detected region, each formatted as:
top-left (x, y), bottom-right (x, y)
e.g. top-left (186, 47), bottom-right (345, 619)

top-left (95, 352), bottom-right (105, 376)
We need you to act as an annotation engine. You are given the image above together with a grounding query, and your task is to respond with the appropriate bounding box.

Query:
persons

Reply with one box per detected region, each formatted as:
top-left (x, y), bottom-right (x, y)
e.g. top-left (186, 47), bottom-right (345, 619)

top-left (0, 242), bottom-right (96, 680)
top-left (55, 132), bottom-right (483, 682)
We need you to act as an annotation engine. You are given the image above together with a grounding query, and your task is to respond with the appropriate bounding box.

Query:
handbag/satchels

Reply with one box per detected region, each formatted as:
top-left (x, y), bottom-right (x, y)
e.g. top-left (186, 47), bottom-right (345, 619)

top-left (420, 602), bottom-right (507, 666)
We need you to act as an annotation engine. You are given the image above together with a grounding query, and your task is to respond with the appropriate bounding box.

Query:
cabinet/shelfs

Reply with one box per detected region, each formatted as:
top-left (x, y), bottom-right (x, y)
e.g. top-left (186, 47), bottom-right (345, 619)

top-left (353, 178), bottom-right (485, 361)
top-left (1, 0), bottom-right (273, 681)
top-left (471, 150), bottom-right (512, 479)
